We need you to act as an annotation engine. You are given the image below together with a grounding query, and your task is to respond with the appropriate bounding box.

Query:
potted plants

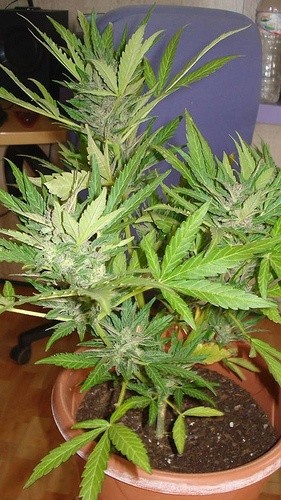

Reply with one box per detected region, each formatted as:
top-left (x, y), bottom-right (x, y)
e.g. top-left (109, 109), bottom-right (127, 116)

top-left (2, 10), bottom-right (279, 498)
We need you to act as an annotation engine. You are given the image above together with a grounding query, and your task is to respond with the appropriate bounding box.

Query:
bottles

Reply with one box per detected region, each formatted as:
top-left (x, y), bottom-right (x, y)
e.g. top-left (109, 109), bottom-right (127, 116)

top-left (255, 0), bottom-right (281, 104)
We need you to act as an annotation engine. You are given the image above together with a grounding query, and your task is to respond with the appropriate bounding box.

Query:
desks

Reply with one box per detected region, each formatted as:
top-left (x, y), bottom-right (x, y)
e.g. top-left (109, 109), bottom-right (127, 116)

top-left (2, 105), bottom-right (71, 147)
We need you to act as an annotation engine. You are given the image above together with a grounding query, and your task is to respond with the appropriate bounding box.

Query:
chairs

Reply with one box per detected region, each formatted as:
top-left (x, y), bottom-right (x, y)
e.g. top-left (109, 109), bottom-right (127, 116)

top-left (87, 3), bottom-right (268, 201)
